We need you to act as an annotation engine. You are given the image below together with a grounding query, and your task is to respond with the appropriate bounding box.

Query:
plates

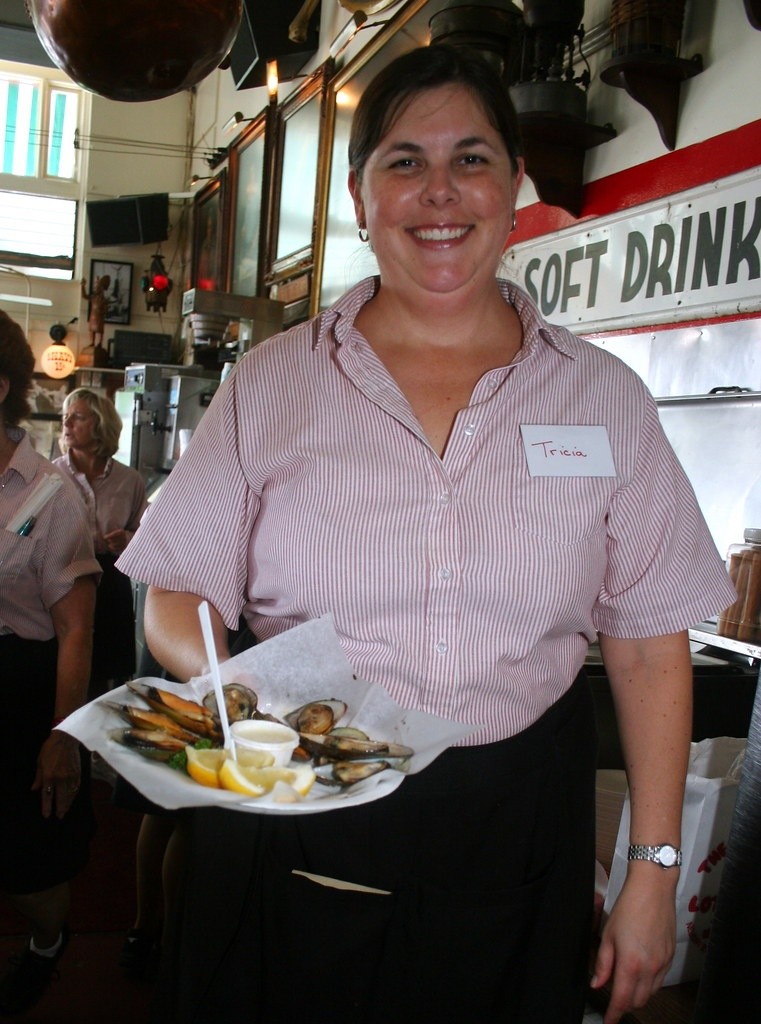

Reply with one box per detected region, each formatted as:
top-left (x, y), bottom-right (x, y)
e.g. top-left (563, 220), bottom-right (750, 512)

top-left (219, 776), bottom-right (406, 816)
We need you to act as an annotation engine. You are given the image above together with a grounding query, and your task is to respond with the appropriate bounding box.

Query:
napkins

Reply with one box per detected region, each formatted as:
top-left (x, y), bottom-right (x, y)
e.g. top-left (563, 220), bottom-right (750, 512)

top-left (52, 611), bottom-right (486, 810)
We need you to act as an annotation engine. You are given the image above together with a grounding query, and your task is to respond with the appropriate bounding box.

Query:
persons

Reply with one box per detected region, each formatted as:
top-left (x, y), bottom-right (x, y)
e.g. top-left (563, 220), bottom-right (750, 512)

top-left (199, 215), bottom-right (216, 280)
top-left (82, 277), bottom-right (107, 348)
top-left (50, 388), bottom-right (149, 788)
top-left (113, 47), bottom-right (740, 1024)
top-left (0, 310), bottom-right (104, 1017)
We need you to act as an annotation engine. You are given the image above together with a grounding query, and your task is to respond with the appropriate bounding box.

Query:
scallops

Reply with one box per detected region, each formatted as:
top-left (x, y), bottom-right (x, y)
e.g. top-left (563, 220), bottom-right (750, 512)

top-left (102, 678), bottom-right (414, 788)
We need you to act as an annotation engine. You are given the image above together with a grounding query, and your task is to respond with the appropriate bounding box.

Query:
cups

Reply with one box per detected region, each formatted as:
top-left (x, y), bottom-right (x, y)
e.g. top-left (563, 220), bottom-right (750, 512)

top-left (229, 719), bottom-right (300, 767)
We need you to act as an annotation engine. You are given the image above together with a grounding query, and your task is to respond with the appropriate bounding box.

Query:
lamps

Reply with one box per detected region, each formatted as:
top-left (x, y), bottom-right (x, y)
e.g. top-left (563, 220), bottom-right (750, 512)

top-left (141, 254), bottom-right (173, 313)
top-left (40, 323), bottom-right (76, 380)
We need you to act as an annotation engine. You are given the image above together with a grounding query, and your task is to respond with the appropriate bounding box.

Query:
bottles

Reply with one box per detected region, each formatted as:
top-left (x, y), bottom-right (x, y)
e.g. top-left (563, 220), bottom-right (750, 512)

top-left (725, 528), bottom-right (761, 574)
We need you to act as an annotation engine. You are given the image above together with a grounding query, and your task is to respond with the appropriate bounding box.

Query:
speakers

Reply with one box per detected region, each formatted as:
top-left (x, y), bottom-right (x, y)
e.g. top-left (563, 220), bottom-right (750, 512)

top-left (87, 192), bottom-right (168, 249)
top-left (228, 0), bottom-right (322, 90)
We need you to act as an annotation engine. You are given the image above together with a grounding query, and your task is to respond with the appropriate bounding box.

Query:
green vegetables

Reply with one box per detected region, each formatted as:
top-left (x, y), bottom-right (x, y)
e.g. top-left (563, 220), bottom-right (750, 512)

top-left (167, 739), bottom-right (213, 775)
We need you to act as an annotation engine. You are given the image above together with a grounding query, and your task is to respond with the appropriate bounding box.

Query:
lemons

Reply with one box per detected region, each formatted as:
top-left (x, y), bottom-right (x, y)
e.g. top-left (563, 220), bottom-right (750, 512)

top-left (218, 757), bottom-right (318, 799)
top-left (184, 744), bottom-right (275, 789)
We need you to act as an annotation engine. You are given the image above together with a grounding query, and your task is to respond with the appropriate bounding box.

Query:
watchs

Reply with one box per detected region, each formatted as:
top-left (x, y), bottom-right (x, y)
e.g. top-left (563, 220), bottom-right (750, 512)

top-left (627, 842), bottom-right (682, 870)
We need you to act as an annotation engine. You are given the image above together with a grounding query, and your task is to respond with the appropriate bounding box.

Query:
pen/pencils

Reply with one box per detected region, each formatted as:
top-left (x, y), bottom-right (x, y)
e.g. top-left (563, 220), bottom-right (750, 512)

top-left (0, 516), bottom-right (36, 568)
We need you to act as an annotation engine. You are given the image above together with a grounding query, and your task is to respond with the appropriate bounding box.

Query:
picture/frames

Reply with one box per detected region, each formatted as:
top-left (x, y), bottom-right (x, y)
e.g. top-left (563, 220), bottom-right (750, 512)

top-left (87, 258), bottom-right (135, 326)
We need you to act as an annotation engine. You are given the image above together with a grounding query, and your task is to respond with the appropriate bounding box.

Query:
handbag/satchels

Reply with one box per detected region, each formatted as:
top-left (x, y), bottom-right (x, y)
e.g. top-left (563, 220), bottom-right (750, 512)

top-left (605, 736), bottom-right (746, 989)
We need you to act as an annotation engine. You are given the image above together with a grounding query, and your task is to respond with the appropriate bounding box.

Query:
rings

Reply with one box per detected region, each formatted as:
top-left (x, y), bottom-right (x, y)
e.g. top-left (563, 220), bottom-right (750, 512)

top-left (43, 786), bottom-right (55, 792)
top-left (68, 784), bottom-right (80, 796)
